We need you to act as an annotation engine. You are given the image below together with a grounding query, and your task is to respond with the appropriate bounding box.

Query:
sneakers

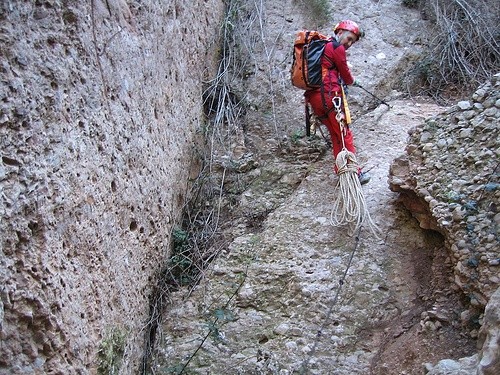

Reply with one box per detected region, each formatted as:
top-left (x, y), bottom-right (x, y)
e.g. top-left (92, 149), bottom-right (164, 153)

top-left (356, 173), bottom-right (371, 184)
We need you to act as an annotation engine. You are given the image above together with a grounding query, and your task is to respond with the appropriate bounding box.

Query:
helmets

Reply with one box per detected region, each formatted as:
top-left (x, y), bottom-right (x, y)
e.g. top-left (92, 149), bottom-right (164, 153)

top-left (334, 20), bottom-right (364, 39)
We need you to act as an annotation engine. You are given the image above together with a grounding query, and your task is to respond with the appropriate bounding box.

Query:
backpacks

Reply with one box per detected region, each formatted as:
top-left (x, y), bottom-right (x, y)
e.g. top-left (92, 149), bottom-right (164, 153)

top-left (291, 28), bottom-right (335, 89)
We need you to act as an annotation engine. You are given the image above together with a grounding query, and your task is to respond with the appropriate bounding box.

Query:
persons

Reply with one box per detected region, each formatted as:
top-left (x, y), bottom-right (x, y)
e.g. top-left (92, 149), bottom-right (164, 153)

top-left (302, 19), bottom-right (372, 186)
